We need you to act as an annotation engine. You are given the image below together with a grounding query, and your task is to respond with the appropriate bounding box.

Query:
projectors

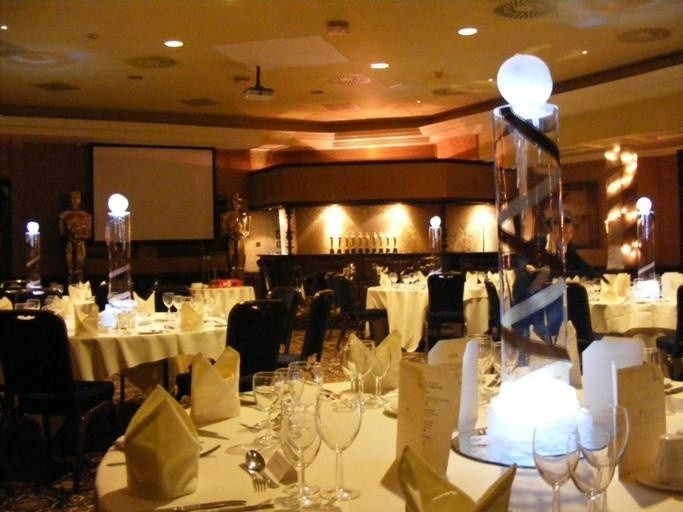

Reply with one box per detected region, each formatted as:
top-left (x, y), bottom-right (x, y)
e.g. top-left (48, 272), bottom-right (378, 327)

top-left (239, 86), bottom-right (274, 102)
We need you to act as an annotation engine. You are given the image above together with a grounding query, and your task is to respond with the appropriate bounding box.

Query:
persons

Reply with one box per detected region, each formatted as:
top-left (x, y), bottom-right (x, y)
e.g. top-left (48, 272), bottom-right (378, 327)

top-left (511, 213), bottom-right (606, 365)
top-left (220, 193), bottom-right (254, 276)
top-left (60, 188), bottom-right (94, 283)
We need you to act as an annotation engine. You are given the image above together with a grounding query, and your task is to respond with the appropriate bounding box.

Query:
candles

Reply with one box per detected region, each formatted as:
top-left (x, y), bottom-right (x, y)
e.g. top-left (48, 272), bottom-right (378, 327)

top-left (326, 233), bottom-right (398, 254)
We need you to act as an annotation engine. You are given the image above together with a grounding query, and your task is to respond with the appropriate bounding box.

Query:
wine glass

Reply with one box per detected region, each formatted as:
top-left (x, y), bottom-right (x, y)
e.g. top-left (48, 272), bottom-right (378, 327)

top-left (531, 401), bottom-right (630, 512)
top-left (161, 291), bottom-right (217, 328)
top-left (388, 268), bottom-right (486, 290)
top-left (250, 358), bottom-right (365, 502)
top-left (339, 339), bottom-right (391, 407)
top-left (465, 332), bottom-right (517, 394)
top-left (113, 302), bottom-right (138, 335)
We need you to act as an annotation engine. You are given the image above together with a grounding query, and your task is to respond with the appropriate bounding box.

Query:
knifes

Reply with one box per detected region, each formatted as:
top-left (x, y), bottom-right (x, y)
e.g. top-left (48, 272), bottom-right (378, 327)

top-left (154, 499), bottom-right (275, 512)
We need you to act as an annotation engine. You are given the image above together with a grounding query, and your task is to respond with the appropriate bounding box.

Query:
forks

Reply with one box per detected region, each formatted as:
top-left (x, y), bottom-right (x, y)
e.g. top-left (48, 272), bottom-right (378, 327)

top-left (239, 460), bottom-right (266, 495)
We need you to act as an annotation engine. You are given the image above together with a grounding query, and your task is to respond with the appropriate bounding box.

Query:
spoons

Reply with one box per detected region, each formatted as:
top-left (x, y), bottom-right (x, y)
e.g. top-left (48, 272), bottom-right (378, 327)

top-left (245, 449), bottom-right (277, 488)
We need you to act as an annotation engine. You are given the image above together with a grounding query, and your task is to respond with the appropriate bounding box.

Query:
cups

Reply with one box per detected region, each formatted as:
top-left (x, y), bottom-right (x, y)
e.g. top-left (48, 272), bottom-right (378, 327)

top-left (581, 276), bottom-right (644, 304)
top-left (14, 298), bottom-right (40, 311)
